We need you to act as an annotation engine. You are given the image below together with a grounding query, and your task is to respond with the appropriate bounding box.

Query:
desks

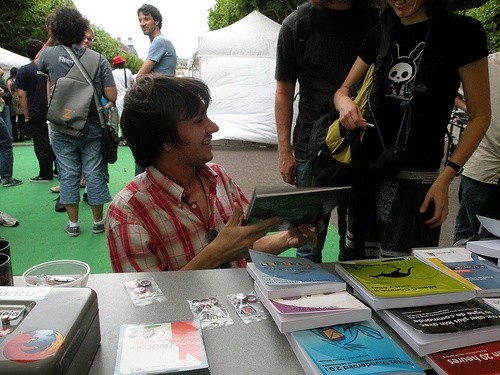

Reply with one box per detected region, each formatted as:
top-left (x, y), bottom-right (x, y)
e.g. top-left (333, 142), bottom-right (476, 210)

top-left (0, 238), bottom-right (500, 375)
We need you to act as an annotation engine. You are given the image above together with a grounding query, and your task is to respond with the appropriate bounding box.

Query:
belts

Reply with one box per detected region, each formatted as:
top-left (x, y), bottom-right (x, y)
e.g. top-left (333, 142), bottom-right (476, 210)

top-left (357, 154), bottom-right (439, 181)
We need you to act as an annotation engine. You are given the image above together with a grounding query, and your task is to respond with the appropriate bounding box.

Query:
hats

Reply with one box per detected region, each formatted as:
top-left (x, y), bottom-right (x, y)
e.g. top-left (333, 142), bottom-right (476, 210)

top-left (112, 56), bottom-right (126, 64)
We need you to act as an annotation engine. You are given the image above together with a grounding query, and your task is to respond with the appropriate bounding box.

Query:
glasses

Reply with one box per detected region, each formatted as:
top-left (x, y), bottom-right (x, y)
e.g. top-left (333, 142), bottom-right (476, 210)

top-left (208, 227), bottom-right (232, 269)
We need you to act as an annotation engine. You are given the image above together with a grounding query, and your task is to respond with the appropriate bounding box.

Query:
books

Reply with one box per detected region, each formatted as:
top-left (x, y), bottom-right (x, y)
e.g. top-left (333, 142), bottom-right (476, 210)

top-left (246, 213), bottom-right (500, 375)
top-left (243, 181), bottom-right (352, 234)
top-left (113, 320), bottom-right (211, 375)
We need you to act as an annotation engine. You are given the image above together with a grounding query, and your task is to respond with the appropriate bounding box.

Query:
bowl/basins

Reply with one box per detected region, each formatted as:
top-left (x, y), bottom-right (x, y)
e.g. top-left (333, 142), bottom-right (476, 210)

top-left (22, 259), bottom-right (91, 288)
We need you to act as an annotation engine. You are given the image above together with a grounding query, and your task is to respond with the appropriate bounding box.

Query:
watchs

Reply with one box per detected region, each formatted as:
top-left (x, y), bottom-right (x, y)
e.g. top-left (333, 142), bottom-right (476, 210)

top-left (443, 159), bottom-right (464, 176)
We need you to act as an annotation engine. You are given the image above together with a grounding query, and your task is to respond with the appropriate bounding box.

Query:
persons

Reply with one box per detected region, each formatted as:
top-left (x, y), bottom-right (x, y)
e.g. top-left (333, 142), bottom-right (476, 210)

top-left (449, 51), bottom-right (500, 246)
top-left (102, 71), bottom-right (326, 274)
top-left (0, 6), bottom-right (119, 239)
top-left (109, 54), bottom-right (136, 147)
top-left (332, 0), bottom-right (492, 262)
top-left (135, 3), bottom-right (177, 80)
top-left (272, 0), bottom-right (383, 264)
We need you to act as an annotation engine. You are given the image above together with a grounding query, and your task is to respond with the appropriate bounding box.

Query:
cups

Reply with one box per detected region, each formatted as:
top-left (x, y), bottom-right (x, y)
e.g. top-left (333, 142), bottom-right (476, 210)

top-left (0, 239), bottom-right (15, 286)
top-left (0, 252), bottom-right (13, 286)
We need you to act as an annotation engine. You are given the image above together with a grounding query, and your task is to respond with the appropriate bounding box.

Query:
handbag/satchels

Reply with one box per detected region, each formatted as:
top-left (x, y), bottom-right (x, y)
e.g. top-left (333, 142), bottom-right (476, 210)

top-left (101, 125), bottom-right (123, 163)
top-left (308, 112), bottom-right (360, 187)
top-left (45, 77), bottom-right (94, 138)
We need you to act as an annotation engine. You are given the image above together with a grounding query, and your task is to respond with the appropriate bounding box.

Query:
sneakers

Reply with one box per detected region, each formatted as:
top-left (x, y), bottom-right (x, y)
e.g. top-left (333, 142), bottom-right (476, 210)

top-left (54, 195), bottom-right (66, 212)
top-left (0, 211), bottom-right (19, 227)
top-left (29, 176), bottom-right (52, 183)
top-left (2, 178), bottom-right (22, 187)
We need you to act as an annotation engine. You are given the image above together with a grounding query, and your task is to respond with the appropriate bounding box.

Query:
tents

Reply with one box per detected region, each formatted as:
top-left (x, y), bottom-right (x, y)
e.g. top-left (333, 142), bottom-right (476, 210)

top-left (195, 9), bottom-right (300, 149)
top-left (0, 47), bottom-right (32, 123)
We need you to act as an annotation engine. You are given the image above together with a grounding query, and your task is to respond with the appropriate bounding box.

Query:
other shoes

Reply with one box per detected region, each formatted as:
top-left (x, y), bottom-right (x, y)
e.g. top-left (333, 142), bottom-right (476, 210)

top-left (118, 141), bottom-right (124, 146)
top-left (19, 137), bottom-right (24, 141)
top-left (93, 223), bottom-right (105, 233)
top-left (123, 140), bottom-right (127, 146)
top-left (12, 137), bottom-right (18, 142)
top-left (83, 192), bottom-right (88, 202)
top-left (53, 169), bottom-right (59, 177)
top-left (24, 136), bottom-right (31, 140)
top-left (50, 186), bottom-right (61, 192)
top-left (64, 223), bottom-right (80, 236)
